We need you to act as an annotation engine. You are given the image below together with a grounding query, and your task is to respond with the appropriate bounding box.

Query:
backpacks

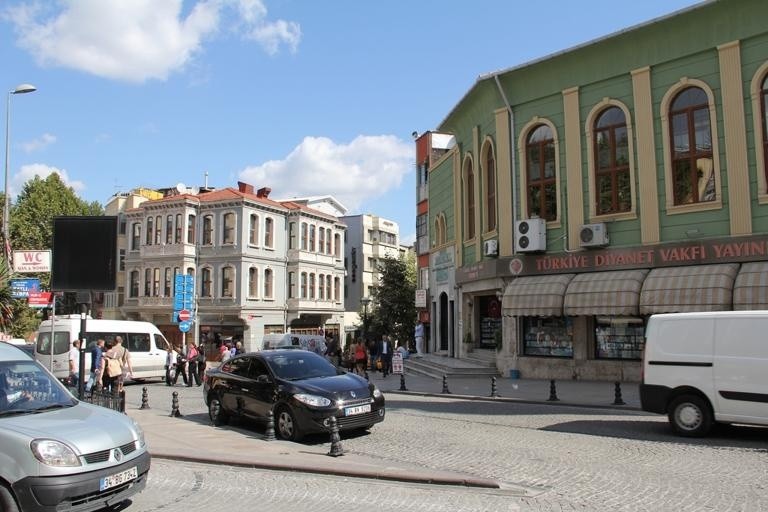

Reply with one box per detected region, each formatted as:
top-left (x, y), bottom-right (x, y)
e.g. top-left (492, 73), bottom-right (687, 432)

top-left (105, 352), bottom-right (122, 378)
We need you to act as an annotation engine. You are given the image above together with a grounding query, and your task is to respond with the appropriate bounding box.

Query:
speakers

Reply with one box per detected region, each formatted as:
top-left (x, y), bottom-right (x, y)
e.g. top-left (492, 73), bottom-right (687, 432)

top-left (51, 215), bottom-right (118, 291)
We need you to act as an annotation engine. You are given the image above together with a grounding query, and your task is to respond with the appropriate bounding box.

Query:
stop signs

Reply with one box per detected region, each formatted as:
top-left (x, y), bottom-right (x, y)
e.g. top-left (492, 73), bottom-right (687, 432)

top-left (179, 310), bottom-right (190, 321)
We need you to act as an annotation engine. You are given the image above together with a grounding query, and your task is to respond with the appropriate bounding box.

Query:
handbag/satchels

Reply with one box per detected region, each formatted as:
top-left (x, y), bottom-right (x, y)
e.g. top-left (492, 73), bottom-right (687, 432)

top-left (67, 374), bottom-right (77, 387)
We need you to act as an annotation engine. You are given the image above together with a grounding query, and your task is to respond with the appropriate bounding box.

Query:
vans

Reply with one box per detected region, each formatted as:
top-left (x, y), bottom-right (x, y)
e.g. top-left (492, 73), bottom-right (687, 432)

top-left (0, 341), bottom-right (148, 512)
top-left (639, 310), bottom-right (768, 437)
top-left (35, 319), bottom-right (180, 384)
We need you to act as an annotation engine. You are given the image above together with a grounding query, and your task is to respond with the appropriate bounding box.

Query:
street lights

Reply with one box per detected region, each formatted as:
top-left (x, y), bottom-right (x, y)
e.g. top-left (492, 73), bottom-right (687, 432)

top-left (4, 85), bottom-right (37, 259)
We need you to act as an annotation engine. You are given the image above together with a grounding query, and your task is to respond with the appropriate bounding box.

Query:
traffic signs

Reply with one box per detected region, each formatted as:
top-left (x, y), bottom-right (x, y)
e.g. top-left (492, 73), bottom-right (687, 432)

top-left (9, 278), bottom-right (40, 299)
top-left (12, 250), bottom-right (52, 273)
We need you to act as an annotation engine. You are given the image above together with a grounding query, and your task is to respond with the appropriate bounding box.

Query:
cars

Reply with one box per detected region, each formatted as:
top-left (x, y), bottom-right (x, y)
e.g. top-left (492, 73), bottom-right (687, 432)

top-left (203, 349), bottom-right (385, 440)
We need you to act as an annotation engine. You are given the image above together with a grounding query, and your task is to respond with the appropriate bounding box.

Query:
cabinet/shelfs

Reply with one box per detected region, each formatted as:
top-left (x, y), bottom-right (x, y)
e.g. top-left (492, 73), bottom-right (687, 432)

top-left (595, 327), bottom-right (644, 359)
top-left (480, 315), bottom-right (502, 348)
top-left (523, 323), bottom-right (573, 357)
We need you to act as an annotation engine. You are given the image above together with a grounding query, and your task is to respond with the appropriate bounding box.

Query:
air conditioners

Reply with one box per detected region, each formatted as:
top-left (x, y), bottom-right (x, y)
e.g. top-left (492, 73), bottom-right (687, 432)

top-left (482, 240), bottom-right (498, 255)
top-left (579, 221), bottom-right (609, 247)
top-left (512, 219), bottom-right (547, 253)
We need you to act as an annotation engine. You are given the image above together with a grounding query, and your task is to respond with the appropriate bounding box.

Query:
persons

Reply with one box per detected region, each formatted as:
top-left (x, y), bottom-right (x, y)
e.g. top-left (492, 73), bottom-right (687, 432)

top-left (68, 336), bottom-right (133, 403)
top-left (0, 373), bottom-right (34, 407)
top-left (302, 320), bottom-right (426, 379)
top-left (166, 339), bottom-right (247, 387)
